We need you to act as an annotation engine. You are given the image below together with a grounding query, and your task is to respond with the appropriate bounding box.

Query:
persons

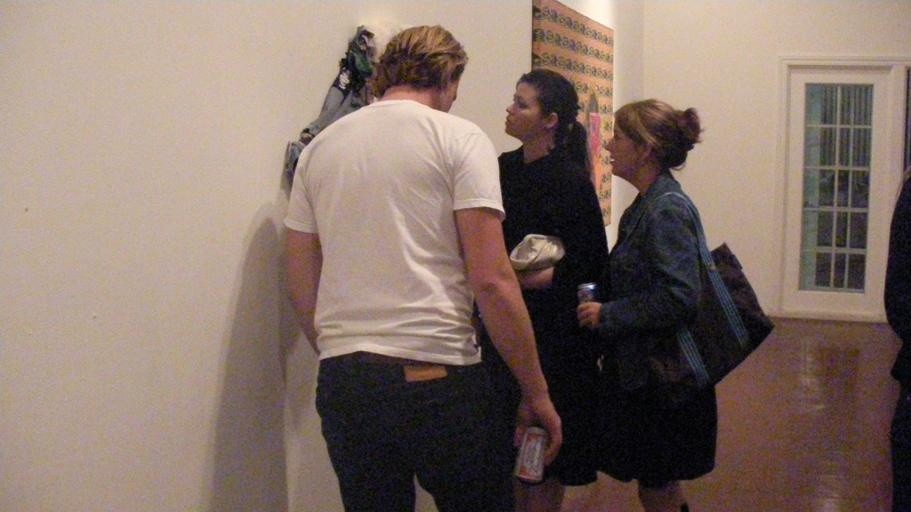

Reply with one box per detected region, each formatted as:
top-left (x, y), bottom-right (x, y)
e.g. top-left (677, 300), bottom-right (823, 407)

top-left (282, 23), bottom-right (563, 511)
top-left (819, 172), bottom-right (845, 264)
top-left (575, 98), bottom-right (717, 512)
top-left (883, 178), bottom-right (911, 512)
top-left (471, 67), bottom-right (609, 511)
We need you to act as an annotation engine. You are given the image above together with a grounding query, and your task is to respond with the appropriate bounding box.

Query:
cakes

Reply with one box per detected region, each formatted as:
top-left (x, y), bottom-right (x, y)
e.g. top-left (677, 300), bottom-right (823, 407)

top-left (577, 282), bottom-right (598, 302)
top-left (513, 426), bottom-right (549, 482)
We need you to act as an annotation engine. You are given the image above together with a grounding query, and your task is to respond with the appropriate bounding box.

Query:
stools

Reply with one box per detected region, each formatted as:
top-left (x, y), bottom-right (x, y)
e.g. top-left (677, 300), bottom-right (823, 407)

top-left (331, 348), bottom-right (426, 368)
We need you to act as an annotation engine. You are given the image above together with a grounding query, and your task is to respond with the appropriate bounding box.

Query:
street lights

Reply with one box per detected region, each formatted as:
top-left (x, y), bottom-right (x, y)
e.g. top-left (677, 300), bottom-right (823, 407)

top-left (633, 243), bottom-right (774, 402)
